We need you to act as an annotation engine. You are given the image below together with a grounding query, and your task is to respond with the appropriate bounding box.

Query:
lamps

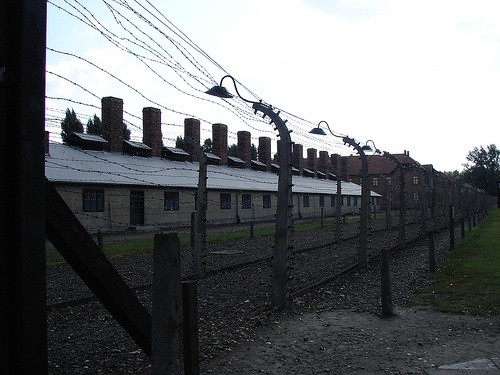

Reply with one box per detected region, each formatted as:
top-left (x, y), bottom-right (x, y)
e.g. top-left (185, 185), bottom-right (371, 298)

top-left (361, 139), bottom-right (384, 154)
top-left (204, 74), bottom-right (258, 105)
top-left (309, 121), bottom-right (345, 140)
